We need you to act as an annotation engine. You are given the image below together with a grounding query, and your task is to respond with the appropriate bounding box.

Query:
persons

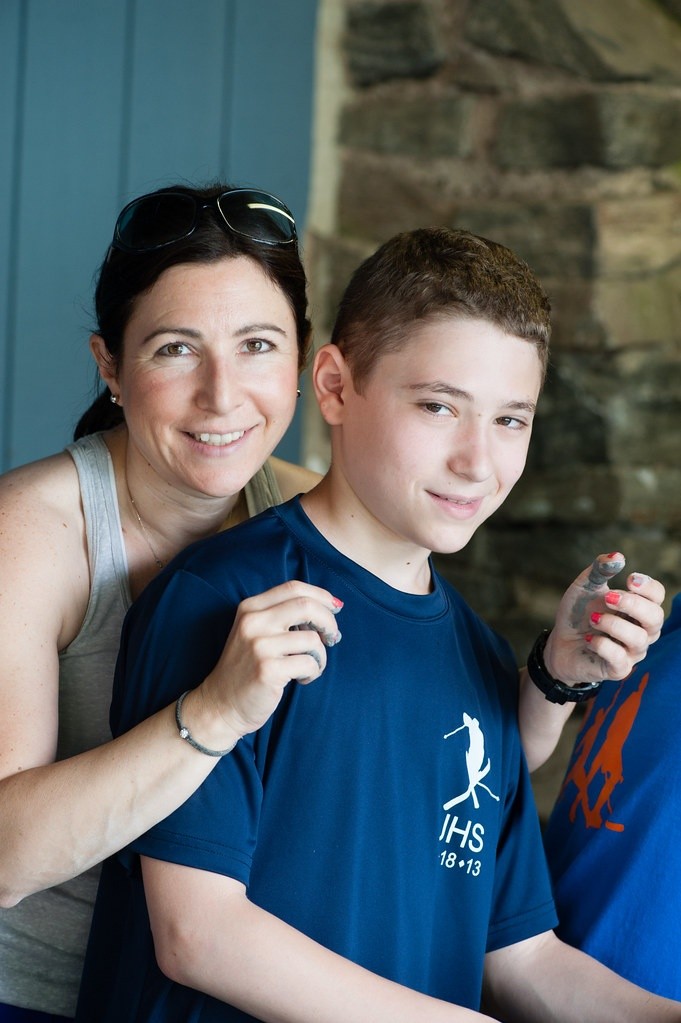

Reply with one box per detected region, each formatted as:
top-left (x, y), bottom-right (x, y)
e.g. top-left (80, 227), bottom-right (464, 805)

top-left (543, 592), bottom-right (681, 1000)
top-left (75, 228), bottom-right (681, 1023)
top-left (0, 182), bottom-right (666, 1023)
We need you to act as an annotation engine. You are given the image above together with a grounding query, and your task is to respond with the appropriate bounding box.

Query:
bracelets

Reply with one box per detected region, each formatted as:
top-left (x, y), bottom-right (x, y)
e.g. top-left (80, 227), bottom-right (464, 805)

top-left (526, 628), bottom-right (604, 706)
top-left (175, 690), bottom-right (237, 757)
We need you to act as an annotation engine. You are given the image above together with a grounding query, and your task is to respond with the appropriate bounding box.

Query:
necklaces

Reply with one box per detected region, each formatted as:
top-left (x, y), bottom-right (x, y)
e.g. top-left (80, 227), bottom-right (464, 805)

top-left (123, 461), bottom-right (166, 570)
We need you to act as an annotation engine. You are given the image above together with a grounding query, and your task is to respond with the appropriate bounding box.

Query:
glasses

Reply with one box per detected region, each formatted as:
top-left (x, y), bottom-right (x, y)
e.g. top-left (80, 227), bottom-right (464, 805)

top-left (98, 188), bottom-right (298, 275)
